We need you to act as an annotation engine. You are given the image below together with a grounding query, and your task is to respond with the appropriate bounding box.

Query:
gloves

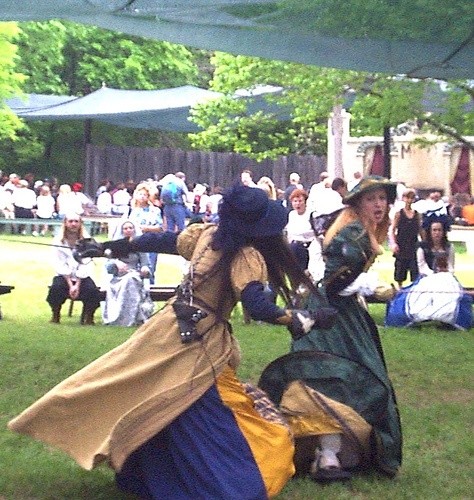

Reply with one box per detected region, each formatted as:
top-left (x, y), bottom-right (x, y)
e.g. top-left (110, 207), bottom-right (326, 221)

top-left (287, 307), bottom-right (339, 340)
top-left (375, 281), bottom-right (400, 301)
top-left (76, 236), bottom-right (130, 259)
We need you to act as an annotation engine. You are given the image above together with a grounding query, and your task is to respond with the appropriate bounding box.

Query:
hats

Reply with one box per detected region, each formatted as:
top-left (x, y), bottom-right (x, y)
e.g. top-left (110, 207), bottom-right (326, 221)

top-left (217, 185), bottom-right (289, 238)
top-left (342, 175), bottom-right (397, 205)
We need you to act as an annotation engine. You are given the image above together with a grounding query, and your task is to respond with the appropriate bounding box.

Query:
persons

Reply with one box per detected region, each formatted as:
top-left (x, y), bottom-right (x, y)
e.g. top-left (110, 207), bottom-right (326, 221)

top-left (47, 212), bottom-right (101, 324)
top-left (76, 185), bottom-right (340, 500)
top-left (0, 169), bottom-right (474, 303)
top-left (386, 218), bottom-right (474, 331)
top-left (100, 221), bottom-right (155, 327)
top-left (258, 176), bottom-right (402, 481)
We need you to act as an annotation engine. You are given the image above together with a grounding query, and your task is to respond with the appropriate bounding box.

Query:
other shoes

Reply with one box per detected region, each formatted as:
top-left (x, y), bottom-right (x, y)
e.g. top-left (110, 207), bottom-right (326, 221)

top-left (50, 315), bottom-right (60, 323)
top-left (311, 467), bottom-right (351, 482)
top-left (81, 318), bottom-right (94, 325)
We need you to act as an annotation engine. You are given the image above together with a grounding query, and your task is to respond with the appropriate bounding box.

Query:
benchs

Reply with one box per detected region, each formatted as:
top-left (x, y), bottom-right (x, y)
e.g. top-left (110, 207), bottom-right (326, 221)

top-left (49, 285), bottom-right (250, 326)
